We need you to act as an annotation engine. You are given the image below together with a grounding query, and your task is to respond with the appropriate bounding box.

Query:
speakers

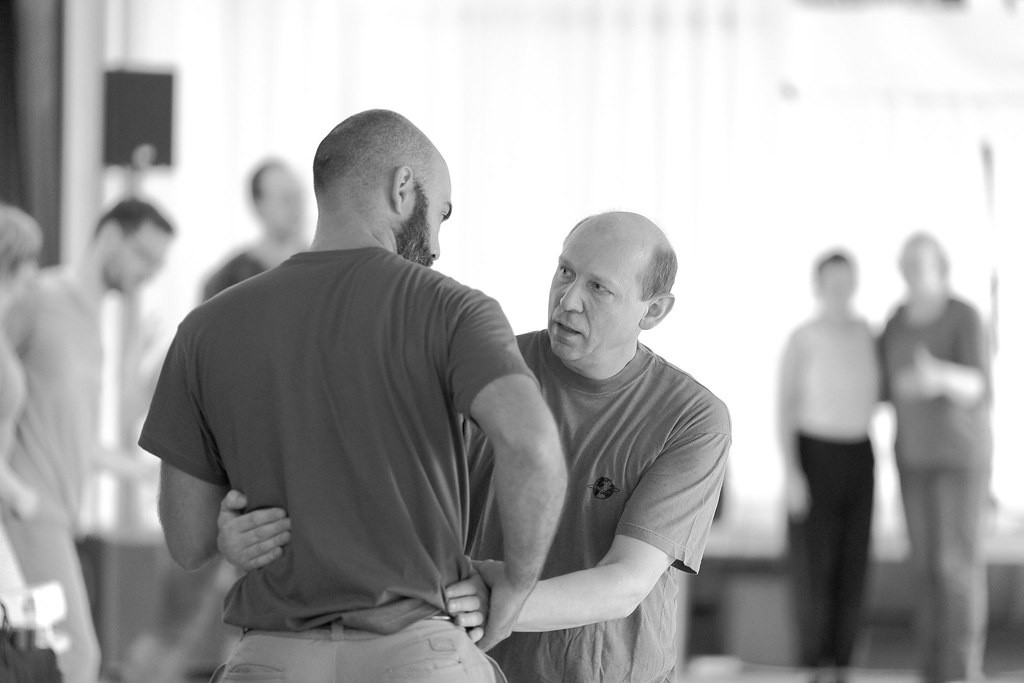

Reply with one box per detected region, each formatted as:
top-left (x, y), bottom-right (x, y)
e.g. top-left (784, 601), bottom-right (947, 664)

top-left (104, 71), bottom-right (174, 168)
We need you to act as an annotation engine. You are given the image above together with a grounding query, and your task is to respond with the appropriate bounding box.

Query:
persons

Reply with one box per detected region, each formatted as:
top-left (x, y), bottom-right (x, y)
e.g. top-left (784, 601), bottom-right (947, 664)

top-left (203, 162), bottom-right (304, 301)
top-left (218, 211), bottom-right (731, 683)
top-left (0, 199), bottom-right (174, 683)
top-left (139, 110), bottom-right (568, 683)
top-left (779, 233), bottom-right (993, 683)
